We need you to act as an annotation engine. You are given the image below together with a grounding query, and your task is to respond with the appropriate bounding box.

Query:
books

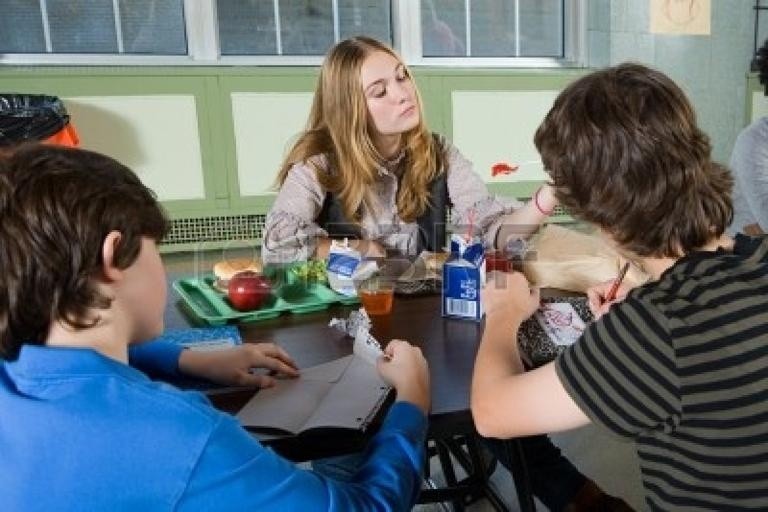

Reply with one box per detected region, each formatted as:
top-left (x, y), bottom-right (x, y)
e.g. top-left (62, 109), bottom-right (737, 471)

top-left (233, 326), bottom-right (392, 441)
top-left (515, 296), bottom-right (597, 370)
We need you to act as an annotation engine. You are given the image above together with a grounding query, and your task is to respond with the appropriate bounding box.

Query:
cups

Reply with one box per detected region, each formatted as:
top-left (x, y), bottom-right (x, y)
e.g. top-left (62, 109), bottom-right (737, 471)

top-left (485, 251), bottom-right (511, 272)
top-left (354, 276), bottom-right (395, 316)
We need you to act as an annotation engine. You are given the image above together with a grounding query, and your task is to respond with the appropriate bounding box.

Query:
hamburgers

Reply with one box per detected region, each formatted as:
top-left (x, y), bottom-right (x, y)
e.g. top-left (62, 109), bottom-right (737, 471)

top-left (213, 260), bottom-right (265, 292)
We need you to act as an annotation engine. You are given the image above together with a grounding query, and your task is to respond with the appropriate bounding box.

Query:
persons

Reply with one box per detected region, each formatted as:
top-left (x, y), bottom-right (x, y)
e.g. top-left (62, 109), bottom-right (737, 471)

top-left (257, 39), bottom-right (558, 263)
top-left (719, 38), bottom-right (767, 239)
top-left (467, 64), bottom-right (768, 512)
top-left (0, 145), bottom-right (432, 512)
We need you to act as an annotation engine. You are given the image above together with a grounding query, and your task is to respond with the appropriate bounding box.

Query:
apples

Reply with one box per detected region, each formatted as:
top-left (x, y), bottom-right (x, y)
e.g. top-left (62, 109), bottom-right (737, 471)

top-left (227, 271), bottom-right (271, 310)
top-left (484, 252), bottom-right (513, 273)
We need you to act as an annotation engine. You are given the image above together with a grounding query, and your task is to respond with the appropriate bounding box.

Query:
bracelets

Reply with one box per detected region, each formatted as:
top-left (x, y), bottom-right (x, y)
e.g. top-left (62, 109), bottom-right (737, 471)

top-left (534, 187), bottom-right (555, 216)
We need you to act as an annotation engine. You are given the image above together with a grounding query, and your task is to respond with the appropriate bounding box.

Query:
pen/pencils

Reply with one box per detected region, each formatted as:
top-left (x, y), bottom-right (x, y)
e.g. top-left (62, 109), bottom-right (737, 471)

top-left (603, 262), bottom-right (631, 302)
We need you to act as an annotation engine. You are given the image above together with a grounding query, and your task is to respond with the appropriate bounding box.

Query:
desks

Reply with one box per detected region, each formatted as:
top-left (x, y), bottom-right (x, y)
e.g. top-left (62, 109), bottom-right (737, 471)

top-left (157, 269), bottom-right (572, 512)
